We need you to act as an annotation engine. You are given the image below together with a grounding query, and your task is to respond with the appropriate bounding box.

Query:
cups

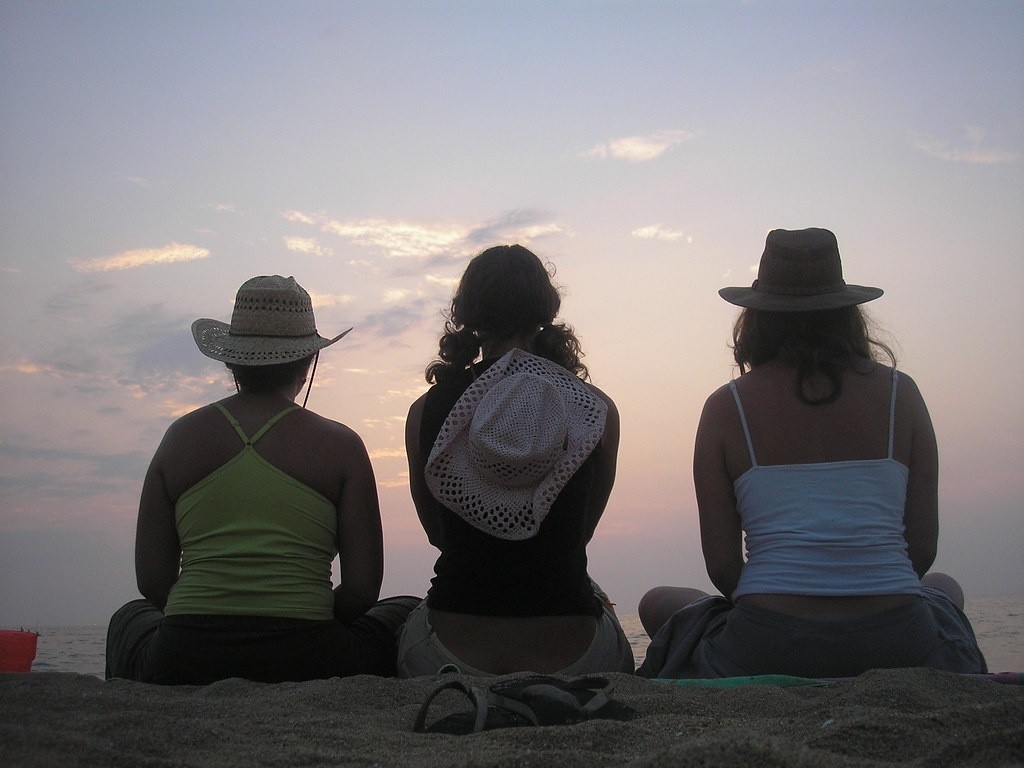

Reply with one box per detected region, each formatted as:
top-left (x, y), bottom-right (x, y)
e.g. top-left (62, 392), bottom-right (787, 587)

top-left (0, 630), bottom-right (41, 675)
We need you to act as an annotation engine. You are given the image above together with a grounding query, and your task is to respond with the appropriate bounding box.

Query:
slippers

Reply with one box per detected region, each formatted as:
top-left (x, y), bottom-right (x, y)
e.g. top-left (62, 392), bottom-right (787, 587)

top-left (491, 671), bottom-right (641, 723)
top-left (415, 680), bottom-right (539, 736)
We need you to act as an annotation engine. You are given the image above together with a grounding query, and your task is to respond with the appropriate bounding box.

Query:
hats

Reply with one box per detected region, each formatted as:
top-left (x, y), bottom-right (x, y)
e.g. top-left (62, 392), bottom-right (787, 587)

top-left (718, 227), bottom-right (882, 311)
top-left (425, 348), bottom-right (608, 540)
top-left (191, 275), bottom-right (355, 366)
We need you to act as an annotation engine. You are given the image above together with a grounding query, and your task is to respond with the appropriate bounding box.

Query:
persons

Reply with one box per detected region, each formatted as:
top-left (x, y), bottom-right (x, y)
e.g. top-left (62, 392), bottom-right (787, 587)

top-left (104, 275), bottom-right (424, 686)
top-left (635, 228), bottom-right (989, 680)
top-left (393, 244), bottom-right (635, 678)
